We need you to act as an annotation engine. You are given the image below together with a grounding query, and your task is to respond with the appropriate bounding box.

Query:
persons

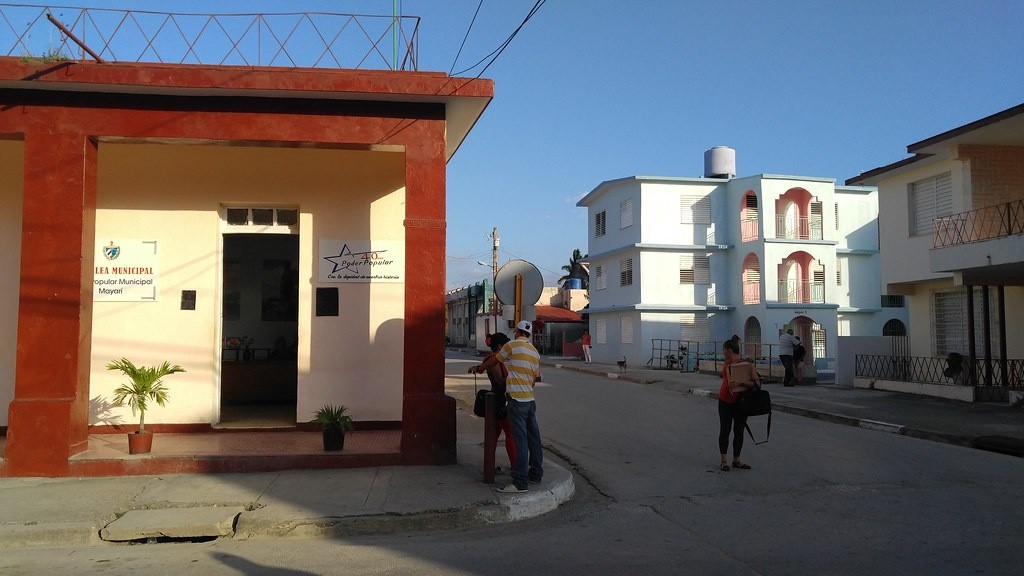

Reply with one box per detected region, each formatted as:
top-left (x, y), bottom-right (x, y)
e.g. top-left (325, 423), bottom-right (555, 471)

top-left (779, 330), bottom-right (801, 387)
top-left (581, 331), bottom-right (593, 364)
top-left (469, 333), bottom-right (542, 474)
top-left (792, 336), bottom-right (805, 385)
top-left (718, 335), bottom-right (761, 472)
top-left (474, 320), bottom-right (544, 493)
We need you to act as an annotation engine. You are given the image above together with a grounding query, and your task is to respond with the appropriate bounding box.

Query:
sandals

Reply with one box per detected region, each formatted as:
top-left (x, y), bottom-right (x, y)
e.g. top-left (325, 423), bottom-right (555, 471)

top-left (721, 461), bottom-right (730, 471)
top-left (732, 461), bottom-right (751, 469)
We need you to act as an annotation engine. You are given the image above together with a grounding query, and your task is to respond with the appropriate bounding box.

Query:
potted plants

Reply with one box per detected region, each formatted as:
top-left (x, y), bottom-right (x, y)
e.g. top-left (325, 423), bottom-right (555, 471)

top-left (105, 357), bottom-right (188, 455)
top-left (314, 404), bottom-right (353, 451)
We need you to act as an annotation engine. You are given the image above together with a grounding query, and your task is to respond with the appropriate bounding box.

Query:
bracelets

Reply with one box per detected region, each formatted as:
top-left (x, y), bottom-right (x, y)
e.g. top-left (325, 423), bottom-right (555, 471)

top-left (476, 365), bottom-right (480, 371)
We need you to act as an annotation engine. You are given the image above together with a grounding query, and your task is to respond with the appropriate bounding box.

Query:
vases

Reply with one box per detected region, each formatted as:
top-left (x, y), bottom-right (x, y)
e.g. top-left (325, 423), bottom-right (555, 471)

top-left (243, 346), bottom-right (251, 361)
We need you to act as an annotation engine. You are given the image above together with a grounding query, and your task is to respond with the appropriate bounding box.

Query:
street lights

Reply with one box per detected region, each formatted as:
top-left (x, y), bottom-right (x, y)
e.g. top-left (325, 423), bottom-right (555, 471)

top-left (477, 260), bottom-right (498, 334)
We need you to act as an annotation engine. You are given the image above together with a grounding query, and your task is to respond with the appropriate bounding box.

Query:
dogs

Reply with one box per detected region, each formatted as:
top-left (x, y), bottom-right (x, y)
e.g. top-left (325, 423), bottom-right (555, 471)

top-left (617, 356), bottom-right (627, 373)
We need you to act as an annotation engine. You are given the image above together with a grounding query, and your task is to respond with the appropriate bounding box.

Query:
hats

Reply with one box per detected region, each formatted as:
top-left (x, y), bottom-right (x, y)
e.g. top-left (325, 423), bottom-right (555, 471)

top-left (515, 320), bottom-right (532, 334)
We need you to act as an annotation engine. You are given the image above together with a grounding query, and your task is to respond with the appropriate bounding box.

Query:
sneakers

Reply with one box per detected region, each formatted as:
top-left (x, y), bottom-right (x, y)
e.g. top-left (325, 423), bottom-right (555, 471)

top-left (529, 474), bottom-right (544, 484)
top-left (496, 481), bottom-right (529, 493)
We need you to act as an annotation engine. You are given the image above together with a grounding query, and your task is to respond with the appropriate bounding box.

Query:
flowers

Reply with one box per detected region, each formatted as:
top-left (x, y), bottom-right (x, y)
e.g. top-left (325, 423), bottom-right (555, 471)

top-left (239, 335), bottom-right (255, 345)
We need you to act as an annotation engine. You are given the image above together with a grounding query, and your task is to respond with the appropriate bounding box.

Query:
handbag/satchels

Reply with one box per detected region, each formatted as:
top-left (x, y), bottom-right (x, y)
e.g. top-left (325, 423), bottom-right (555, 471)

top-left (474, 389), bottom-right (496, 418)
top-left (731, 389), bottom-right (772, 444)
top-left (726, 360), bottom-right (753, 392)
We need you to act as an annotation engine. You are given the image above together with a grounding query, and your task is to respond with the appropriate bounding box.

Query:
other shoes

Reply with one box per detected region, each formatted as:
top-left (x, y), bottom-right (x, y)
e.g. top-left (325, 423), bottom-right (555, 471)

top-left (584, 362), bottom-right (592, 364)
